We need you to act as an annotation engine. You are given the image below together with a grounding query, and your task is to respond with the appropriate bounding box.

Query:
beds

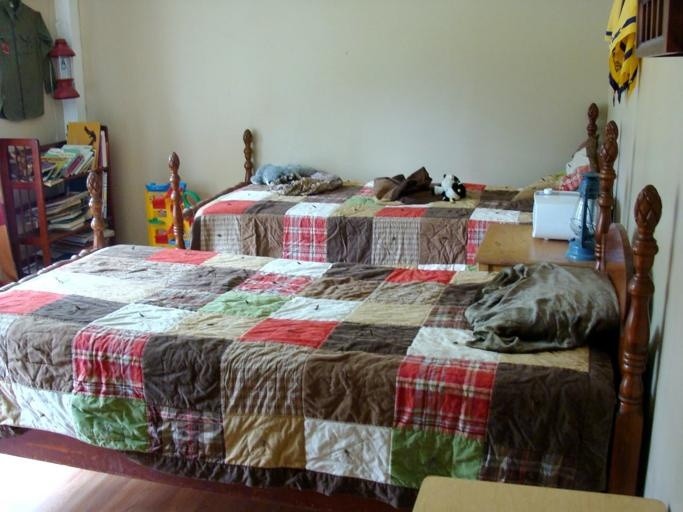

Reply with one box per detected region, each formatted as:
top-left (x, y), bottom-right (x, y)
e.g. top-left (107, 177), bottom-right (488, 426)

top-left (175, 101), bottom-right (610, 261)
top-left (2, 119), bottom-right (662, 436)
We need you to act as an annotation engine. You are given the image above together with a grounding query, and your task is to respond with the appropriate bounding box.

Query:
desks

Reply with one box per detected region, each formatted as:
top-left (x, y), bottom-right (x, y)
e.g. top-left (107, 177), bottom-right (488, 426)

top-left (474, 221), bottom-right (595, 268)
top-left (413, 476), bottom-right (673, 511)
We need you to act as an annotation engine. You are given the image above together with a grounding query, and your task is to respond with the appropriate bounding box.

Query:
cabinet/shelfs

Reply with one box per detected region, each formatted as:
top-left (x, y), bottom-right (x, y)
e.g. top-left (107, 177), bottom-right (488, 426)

top-left (2, 121), bottom-right (109, 278)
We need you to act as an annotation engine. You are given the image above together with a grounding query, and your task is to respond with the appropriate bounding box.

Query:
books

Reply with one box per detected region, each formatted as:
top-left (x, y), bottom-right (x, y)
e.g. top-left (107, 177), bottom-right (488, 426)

top-left (9, 122), bottom-right (115, 274)
